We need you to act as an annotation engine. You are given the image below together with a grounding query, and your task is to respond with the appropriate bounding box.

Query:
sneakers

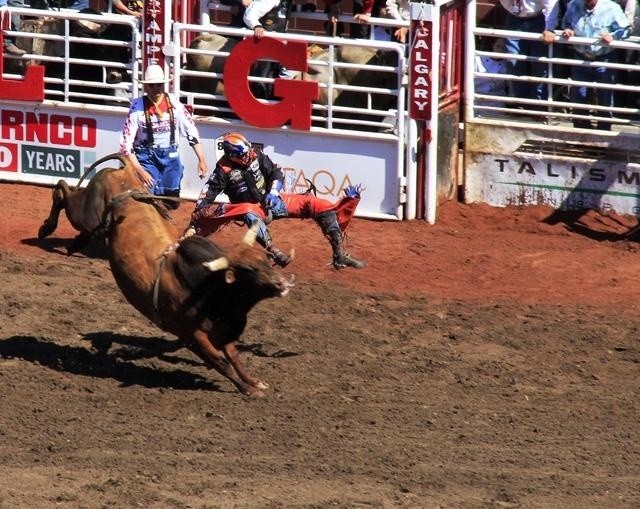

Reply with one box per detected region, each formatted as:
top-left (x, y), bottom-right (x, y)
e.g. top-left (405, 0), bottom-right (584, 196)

top-left (3, 43), bottom-right (27, 55)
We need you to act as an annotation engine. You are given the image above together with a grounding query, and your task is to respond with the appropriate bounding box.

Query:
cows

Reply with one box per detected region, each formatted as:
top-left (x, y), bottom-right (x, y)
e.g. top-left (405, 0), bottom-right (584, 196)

top-left (65, 6), bottom-right (133, 106)
top-left (35, 150), bottom-right (296, 402)
top-left (185, 31), bottom-right (387, 125)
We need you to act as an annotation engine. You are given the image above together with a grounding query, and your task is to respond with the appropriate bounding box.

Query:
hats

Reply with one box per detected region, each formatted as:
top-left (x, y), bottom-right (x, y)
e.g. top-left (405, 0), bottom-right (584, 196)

top-left (134, 64), bottom-right (174, 85)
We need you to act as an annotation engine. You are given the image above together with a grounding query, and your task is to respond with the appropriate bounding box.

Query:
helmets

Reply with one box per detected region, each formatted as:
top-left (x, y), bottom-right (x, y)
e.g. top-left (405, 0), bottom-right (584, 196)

top-left (222, 132), bottom-right (251, 157)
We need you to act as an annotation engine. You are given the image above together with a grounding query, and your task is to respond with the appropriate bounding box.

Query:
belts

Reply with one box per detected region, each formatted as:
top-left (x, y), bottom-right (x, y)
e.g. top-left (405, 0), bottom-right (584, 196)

top-left (508, 11), bottom-right (544, 21)
top-left (572, 48), bottom-right (613, 60)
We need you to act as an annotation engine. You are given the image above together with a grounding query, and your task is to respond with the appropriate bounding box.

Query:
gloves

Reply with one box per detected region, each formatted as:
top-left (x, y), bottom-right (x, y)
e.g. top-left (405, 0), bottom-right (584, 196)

top-left (265, 179), bottom-right (284, 208)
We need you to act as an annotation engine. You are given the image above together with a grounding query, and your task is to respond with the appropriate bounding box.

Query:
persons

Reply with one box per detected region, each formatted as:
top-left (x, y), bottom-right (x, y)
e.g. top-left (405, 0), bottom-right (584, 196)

top-left (3, 2), bottom-right (144, 108)
top-left (184, 132), bottom-right (364, 270)
top-left (185, 2), bottom-right (413, 128)
top-left (474, 2), bottom-right (638, 129)
top-left (122, 67), bottom-right (208, 210)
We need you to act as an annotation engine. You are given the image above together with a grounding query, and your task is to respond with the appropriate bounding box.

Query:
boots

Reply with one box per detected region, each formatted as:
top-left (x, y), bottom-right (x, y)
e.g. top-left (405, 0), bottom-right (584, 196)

top-left (327, 229), bottom-right (368, 270)
top-left (255, 228), bottom-right (291, 268)
